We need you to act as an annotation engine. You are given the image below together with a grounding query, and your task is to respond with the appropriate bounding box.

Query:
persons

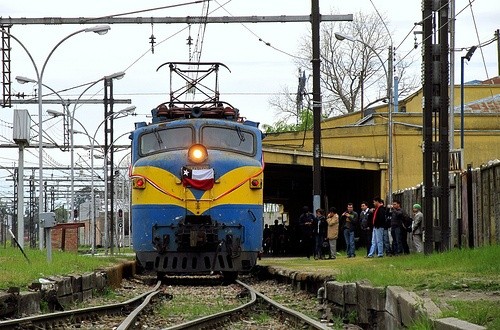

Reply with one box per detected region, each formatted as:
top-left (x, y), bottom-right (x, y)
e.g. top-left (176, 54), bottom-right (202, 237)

top-left (367, 196), bottom-right (385, 258)
top-left (263, 200), bottom-right (424, 260)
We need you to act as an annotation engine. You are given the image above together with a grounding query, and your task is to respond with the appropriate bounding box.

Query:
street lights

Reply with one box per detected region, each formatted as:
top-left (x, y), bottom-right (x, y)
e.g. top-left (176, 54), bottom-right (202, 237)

top-left (0, 25), bottom-right (111, 253)
top-left (68, 129), bottom-right (111, 253)
top-left (334, 33), bottom-right (394, 233)
top-left (16, 73), bottom-right (125, 222)
top-left (45, 106), bottom-right (136, 254)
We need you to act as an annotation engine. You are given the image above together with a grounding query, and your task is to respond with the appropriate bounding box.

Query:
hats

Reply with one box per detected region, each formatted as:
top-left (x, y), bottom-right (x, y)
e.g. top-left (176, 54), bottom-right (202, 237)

top-left (413, 204), bottom-right (421, 210)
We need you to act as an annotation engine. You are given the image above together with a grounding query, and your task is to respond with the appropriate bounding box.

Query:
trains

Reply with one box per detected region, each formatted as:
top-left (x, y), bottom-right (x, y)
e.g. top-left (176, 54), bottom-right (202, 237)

top-left (130, 62), bottom-right (264, 275)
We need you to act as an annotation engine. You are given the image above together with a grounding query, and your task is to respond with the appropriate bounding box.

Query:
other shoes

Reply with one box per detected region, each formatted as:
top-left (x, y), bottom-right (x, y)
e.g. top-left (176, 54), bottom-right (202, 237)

top-left (345, 254), bottom-right (355, 258)
top-left (388, 252), bottom-right (393, 257)
